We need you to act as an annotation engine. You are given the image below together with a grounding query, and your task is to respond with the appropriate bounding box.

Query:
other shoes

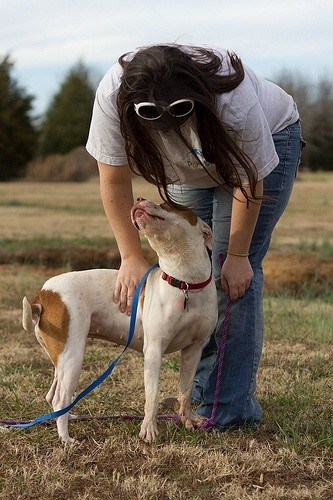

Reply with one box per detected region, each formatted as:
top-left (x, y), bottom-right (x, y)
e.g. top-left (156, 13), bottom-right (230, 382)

top-left (161, 397), bottom-right (213, 428)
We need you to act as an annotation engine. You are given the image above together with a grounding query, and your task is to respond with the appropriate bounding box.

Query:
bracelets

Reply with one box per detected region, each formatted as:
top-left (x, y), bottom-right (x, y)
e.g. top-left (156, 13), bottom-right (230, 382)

top-left (227, 250), bottom-right (249, 257)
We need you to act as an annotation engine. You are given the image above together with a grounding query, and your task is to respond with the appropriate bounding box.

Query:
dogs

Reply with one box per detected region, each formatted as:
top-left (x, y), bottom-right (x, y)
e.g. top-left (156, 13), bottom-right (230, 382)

top-left (21, 197), bottom-right (218, 447)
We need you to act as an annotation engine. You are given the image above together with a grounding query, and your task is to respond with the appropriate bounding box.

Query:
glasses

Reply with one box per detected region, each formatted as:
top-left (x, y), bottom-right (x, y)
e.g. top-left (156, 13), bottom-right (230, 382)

top-left (134, 98), bottom-right (194, 120)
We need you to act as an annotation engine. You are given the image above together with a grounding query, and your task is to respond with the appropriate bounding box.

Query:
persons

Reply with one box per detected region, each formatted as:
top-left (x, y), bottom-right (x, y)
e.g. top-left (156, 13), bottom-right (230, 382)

top-left (84, 42), bottom-right (307, 432)
